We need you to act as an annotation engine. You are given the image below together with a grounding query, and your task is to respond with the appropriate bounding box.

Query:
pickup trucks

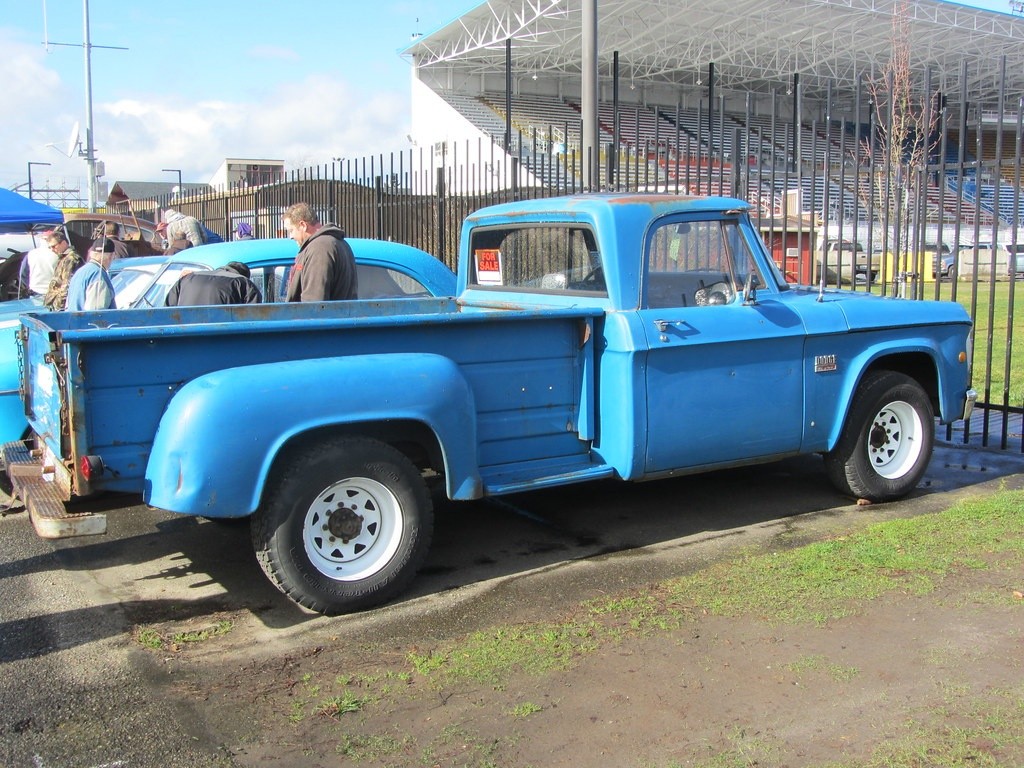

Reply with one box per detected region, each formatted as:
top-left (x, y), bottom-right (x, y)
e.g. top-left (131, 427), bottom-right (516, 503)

top-left (1, 190), bottom-right (978, 619)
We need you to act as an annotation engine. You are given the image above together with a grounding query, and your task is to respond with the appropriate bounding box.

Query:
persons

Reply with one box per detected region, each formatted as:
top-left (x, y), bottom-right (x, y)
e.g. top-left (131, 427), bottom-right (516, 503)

top-left (67, 237), bottom-right (117, 311)
top-left (282, 202), bottom-right (359, 303)
top-left (164, 262), bottom-right (262, 308)
top-left (20, 209), bottom-right (255, 311)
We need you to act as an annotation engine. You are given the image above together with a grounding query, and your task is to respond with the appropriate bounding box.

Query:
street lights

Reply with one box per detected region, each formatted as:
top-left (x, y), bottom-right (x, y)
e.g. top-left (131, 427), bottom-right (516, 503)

top-left (28, 162), bottom-right (51, 200)
top-left (162, 169), bottom-right (181, 214)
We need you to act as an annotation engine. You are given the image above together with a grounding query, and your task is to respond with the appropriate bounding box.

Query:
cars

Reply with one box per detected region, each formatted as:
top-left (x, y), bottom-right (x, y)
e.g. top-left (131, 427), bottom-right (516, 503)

top-left (0, 238), bottom-right (456, 470)
top-left (923, 241), bottom-right (1024, 279)
top-left (45, 213), bottom-right (163, 263)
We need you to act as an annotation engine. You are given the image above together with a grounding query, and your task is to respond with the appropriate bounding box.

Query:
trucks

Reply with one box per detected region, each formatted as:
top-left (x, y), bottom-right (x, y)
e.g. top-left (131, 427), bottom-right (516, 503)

top-left (815, 238), bottom-right (881, 284)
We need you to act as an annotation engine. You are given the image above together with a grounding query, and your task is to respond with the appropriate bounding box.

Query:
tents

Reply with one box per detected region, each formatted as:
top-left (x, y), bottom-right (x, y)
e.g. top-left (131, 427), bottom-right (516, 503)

top-left (0, 187), bottom-right (64, 250)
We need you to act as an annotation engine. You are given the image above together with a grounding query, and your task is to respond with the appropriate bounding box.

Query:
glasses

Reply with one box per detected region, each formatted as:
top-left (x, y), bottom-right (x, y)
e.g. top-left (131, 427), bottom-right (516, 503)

top-left (48, 242), bottom-right (59, 250)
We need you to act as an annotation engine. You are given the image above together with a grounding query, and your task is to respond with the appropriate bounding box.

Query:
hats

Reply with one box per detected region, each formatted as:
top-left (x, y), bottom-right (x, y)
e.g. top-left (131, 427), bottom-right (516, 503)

top-left (155, 222), bottom-right (168, 232)
top-left (92, 238), bottom-right (115, 252)
top-left (165, 209), bottom-right (178, 224)
